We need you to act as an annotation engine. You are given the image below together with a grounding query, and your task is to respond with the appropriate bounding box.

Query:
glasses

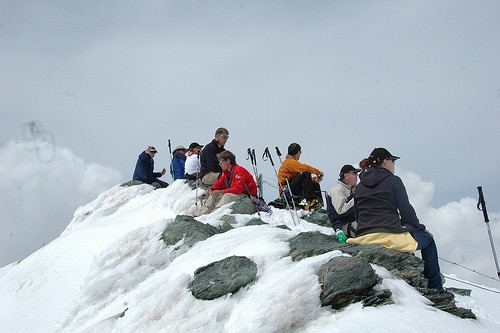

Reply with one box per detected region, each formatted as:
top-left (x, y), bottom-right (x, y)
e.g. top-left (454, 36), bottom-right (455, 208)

top-left (392, 160), bottom-right (394, 163)
top-left (220, 136), bottom-right (229, 139)
top-left (150, 151), bottom-right (157, 153)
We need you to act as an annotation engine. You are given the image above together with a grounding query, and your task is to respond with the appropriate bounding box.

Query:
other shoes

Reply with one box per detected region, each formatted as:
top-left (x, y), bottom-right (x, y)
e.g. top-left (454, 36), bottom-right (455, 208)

top-left (305, 198), bottom-right (324, 212)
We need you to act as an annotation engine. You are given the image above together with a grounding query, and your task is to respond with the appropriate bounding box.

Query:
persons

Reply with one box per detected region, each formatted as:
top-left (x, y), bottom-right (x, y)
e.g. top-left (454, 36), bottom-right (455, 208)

top-left (199, 127), bottom-right (231, 186)
top-left (169, 145), bottom-right (187, 180)
top-left (326, 164), bottom-right (361, 237)
top-left (346, 148), bottom-right (455, 301)
top-left (185, 142), bottom-right (204, 179)
top-left (276, 143), bottom-right (323, 210)
top-left (132, 145), bottom-right (169, 187)
top-left (194, 150), bottom-right (257, 218)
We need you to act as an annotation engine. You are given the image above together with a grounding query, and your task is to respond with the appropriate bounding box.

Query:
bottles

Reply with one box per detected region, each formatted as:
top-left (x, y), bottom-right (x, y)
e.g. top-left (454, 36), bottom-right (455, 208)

top-left (336, 229), bottom-right (348, 244)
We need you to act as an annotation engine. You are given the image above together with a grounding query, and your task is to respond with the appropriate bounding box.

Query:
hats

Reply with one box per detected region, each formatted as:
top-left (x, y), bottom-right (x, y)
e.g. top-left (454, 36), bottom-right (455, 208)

top-left (176, 145), bottom-right (189, 151)
top-left (339, 165), bottom-right (361, 176)
top-left (368, 147), bottom-right (400, 161)
top-left (288, 143), bottom-right (301, 156)
top-left (189, 142), bottom-right (204, 148)
